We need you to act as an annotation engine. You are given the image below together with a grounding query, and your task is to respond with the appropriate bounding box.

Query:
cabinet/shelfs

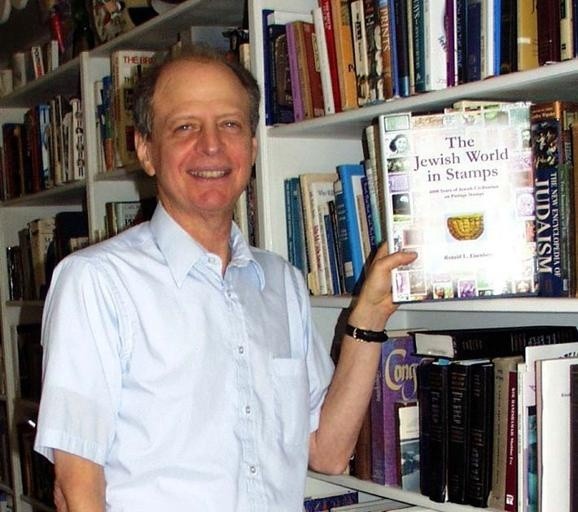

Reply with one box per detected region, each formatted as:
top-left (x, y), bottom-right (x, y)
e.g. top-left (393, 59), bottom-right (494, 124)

top-left (0, 0), bottom-right (578, 512)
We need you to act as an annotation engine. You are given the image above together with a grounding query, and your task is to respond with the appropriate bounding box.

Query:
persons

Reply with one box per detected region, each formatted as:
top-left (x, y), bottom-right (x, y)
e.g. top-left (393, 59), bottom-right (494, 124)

top-left (34, 38), bottom-right (419, 511)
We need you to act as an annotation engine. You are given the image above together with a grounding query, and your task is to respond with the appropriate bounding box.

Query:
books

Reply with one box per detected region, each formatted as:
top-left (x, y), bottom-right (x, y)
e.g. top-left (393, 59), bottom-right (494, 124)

top-left (263, 1), bottom-right (577, 124)
top-left (3, 26), bottom-right (247, 199)
top-left (303, 491), bottom-right (434, 512)
top-left (2, 2), bottom-right (98, 93)
top-left (1, 321), bottom-right (58, 512)
top-left (342, 324), bottom-right (577, 512)
top-left (6, 180), bottom-right (257, 300)
top-left (285, 101), bottom-right (577, 299)
